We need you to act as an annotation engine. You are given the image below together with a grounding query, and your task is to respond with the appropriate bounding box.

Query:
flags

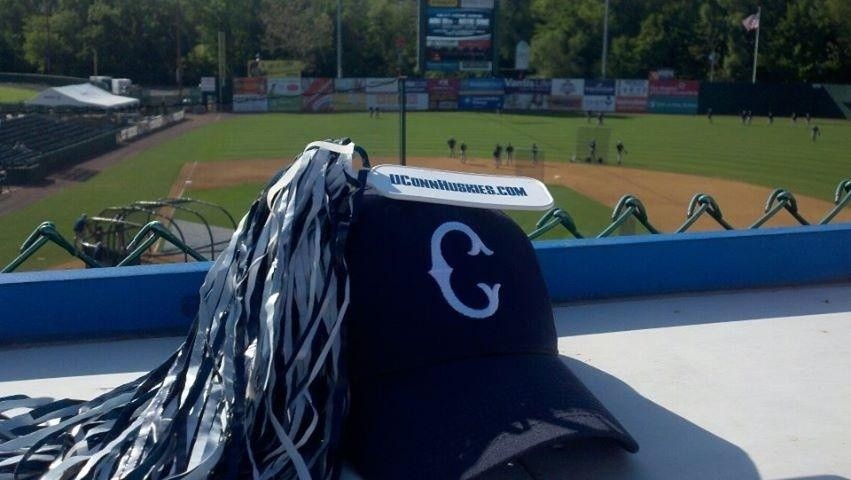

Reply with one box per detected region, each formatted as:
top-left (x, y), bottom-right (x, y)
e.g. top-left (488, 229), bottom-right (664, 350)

top-left (742, 10), bottom-right (759, 31)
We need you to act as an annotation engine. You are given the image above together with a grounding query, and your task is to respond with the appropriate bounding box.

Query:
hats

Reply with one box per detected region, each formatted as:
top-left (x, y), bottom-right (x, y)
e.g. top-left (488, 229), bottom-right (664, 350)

top-left (336, 197), bottom-right (638, 480)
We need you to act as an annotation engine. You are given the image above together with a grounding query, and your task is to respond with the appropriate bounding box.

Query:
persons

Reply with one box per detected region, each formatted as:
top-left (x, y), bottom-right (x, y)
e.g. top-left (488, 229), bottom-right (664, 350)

top-left (586, 107), bottom-right (605, 128)
top-left (531, 142), bottom-right (538, 163)
top-left (741, 108), bottom-right (753, 126)
top-left (588, 136), bottom-right (597, 160)
top-left (460, 141), bottom-right (468, 163)
top-left (616, 140), bottom-right (629, 164)
top-left (492, 140), bottom-right (514, 170)
top-left (791, 112), bottom-right (822, 142)
top-left (766, 109), bottom-right (775, 127)
top-left (446, 135), bottom-right (456, 159)
top-left (707, 104), bottom-right (713, 123)
top-left (368, 104), bottom-right (381, 119)
